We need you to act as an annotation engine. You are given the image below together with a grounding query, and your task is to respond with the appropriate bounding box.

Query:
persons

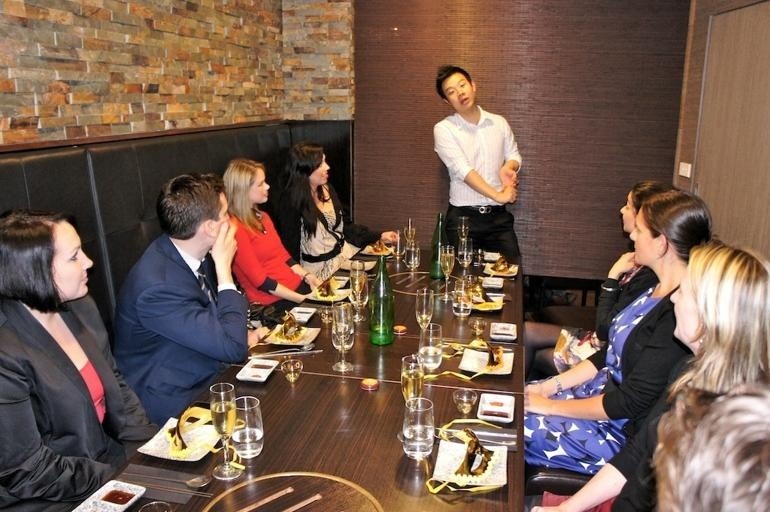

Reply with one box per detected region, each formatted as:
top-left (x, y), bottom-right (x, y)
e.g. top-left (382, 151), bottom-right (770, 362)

top-left (269, 142), bottom-right (400, 288)
top-left (113, 171), bottom-right (271, 431)
top-left (220, 156), bottom-right (323, 330)
top-left (523, 190), bottom-right (717, 477)
top-left (1, 209), bottom-right (162, 512)
top-left (433, 65), bottom-right (522, 258)
top-left (525, 178), bottom-right (678, 384)
top-left (532, 239), bottom-right (770, 511)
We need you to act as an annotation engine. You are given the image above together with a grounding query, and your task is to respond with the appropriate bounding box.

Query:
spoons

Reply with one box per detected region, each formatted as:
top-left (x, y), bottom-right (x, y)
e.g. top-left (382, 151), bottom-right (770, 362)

top-left (257, 343), bottom-right (315, 355)
top-left (450, 343), bottom-right (513, 351)
top-left (122, 471), bottom-right (212, 488)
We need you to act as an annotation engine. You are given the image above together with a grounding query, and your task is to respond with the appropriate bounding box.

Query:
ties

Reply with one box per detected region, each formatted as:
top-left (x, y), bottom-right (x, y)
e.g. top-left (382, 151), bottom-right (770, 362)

top-left (195, 266), bottom-right (216, 308)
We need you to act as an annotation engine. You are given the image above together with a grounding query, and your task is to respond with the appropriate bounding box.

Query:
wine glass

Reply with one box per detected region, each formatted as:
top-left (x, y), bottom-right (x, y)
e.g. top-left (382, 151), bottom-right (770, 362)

top-left (332, 302), bottom-right (354, 372)
top-left (439, 244), bottom-right (456, 301)
top-left (348, 271), bottom-right (369, 322)
top-left (456, 215), bottom-right (473, 278)
top-left (209, 382), bottom-right (242, 481)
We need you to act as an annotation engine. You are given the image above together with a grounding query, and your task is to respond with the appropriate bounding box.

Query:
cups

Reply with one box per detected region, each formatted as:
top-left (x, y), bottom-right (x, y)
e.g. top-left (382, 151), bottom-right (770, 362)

top-left (392, 228), bottom-right (421, 271)
top-left (394, 453), bottom-right (433, 497)
top-left (401, 288), bottom-right (444, 459)
top-left (468, 317), bottom-right (487, 337)
top-left (281, 359), bottom-right (303, 382)
top-left (452, 389), bottom-right (478, 414)
top-left (230, 395), bottom-right (264, 459)
top-left (452, 278), bottom-right (473, 317)
top-left (137, 500), bottom-right (172, 512)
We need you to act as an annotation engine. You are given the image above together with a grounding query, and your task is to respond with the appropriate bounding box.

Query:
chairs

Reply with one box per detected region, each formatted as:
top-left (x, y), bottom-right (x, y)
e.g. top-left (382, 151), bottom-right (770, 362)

top-left (526, 285), bottom-right (649, 512)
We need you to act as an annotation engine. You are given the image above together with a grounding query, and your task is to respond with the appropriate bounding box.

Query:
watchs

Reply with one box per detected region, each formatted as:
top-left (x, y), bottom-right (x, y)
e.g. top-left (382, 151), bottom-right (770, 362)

top-left (550, 376), bottom-right (565, 396)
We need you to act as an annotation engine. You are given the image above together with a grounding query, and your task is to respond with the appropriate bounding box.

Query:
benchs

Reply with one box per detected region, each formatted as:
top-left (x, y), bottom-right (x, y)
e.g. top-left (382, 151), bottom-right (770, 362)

top-left (0, 116), bottom-right (357, 364)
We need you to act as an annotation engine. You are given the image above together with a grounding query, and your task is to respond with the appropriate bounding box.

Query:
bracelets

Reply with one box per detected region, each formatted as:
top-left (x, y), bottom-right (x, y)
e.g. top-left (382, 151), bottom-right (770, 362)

top-left (602, 284), bottom-right (620, 293)
top-left (302, 272), bottom-right (310, 281)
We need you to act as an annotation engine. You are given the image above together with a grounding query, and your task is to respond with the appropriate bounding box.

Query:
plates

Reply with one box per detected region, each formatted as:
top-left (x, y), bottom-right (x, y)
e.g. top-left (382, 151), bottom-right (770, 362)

top-left (236, 358), bottom-right (279, 382)
top-left (489, 322), bottom-right (518, 341)
top-left (264, 324), bottom-right (321, 346)
top-left (471, 252), bottom-right (519, 310)
top-left (476, 392), bottom-right (515, 422)
top-left (458, 348), bottom-right (514, 375)
top-left (137, 417), bottom-right (223, 462)
top-left (432, 439), bottom-right (509, 487)
top-left (284, 306), bottom-right (317, 323)
top-left (306, 243), bottom-right (395, 302)
top-left (72, 479), bottom-right (147, 512)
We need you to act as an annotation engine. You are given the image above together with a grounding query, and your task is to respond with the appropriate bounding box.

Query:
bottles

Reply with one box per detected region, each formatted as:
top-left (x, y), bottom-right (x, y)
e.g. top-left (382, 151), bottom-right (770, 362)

top-left (369, 255), bottom-right (395, 345)
top-left (430, 213), bottom-right (448, 279)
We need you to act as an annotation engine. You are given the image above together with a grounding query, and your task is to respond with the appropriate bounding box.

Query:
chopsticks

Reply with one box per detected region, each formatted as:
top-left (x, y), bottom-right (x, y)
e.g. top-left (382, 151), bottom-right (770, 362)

top-left (127, 481), bottom-right (215, 499)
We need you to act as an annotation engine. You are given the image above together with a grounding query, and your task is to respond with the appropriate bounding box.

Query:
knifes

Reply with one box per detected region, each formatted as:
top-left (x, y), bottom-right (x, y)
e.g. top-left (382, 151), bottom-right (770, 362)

top-left (436, 426), bottom-right (518, 439)
top-left (247, 350), bottom-right (324, 359)
